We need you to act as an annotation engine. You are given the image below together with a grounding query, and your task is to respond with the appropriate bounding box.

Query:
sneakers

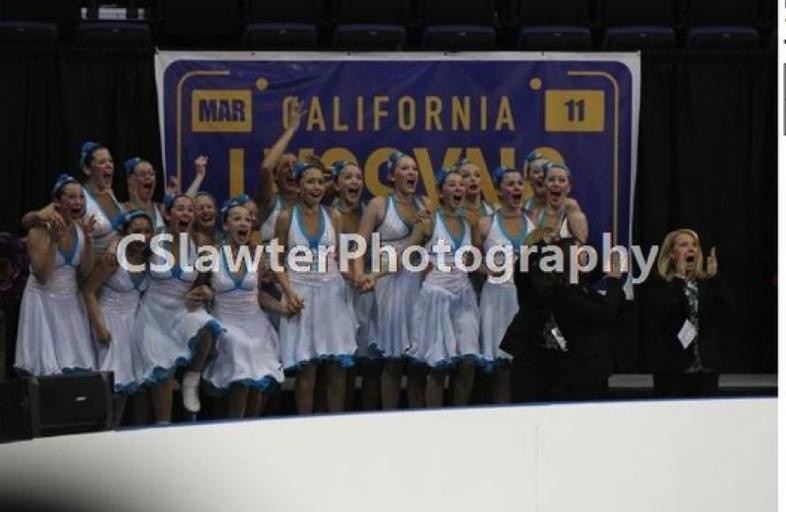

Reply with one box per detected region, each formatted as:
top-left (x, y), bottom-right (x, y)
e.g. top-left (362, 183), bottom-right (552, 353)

top-left (182, 372), bottom-right (201, 413)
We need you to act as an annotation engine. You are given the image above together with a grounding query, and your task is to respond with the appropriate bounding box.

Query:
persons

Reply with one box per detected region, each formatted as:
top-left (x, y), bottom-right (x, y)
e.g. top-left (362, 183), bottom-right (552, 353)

top-left (10, 97), bottom-right (630, 443)
top-left (636, 227), bottom-right (737, 399)
